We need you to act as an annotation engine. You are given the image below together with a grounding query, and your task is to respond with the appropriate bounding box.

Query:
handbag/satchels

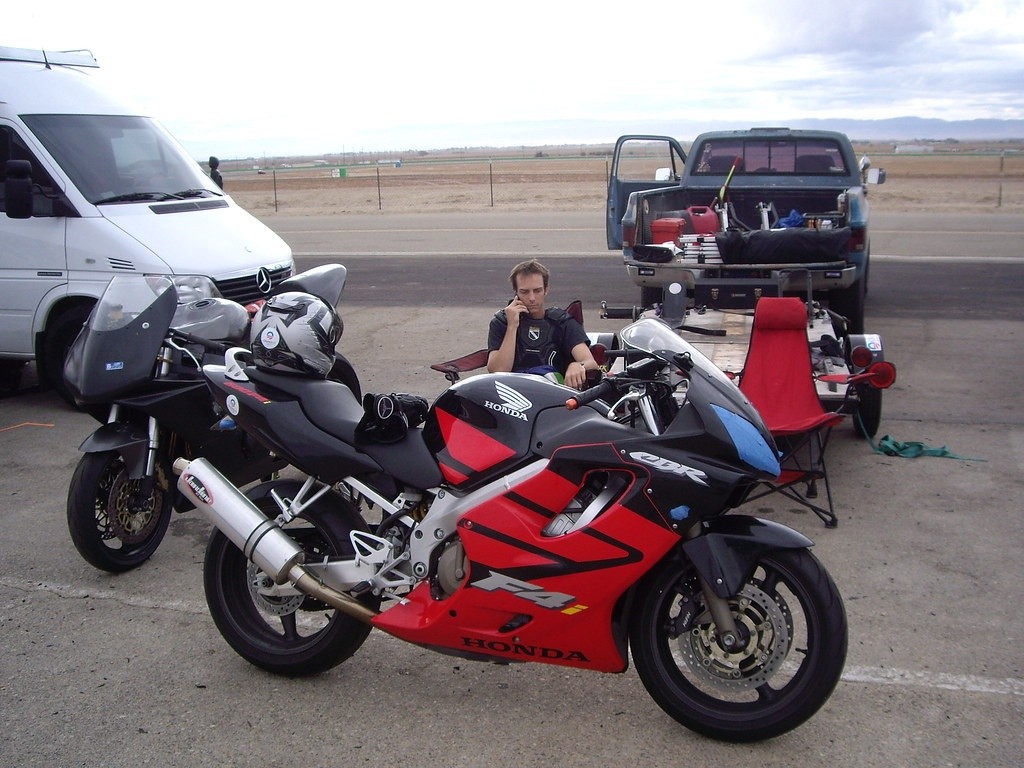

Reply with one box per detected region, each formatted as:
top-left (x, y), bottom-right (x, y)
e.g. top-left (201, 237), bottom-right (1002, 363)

top-left (632, 244), bottom-right (673, 262)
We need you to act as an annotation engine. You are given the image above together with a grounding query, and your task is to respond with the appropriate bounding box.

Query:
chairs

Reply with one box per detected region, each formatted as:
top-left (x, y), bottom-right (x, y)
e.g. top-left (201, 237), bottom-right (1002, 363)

top-left (675, 297), bottom-right (879, 528)
top-left (430, 300), bottom-right (600, 385)
top-left (796, 154), bottom-right (834, 173)
top-left (707, 156), bottom-right (745, 170)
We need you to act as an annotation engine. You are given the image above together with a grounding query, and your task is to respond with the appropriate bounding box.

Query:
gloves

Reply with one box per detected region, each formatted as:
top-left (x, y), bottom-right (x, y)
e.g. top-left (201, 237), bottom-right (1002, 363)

top-left (355, 392), bottom-right (429, 444)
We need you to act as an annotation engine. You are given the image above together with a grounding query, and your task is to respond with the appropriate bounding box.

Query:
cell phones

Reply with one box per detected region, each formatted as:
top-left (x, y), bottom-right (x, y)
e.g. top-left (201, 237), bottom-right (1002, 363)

top-left (517, 297), bottom-right (525, 321)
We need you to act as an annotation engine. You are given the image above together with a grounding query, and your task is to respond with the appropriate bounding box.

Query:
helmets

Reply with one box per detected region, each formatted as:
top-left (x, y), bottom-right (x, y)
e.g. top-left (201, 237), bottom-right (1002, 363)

top-left (250, 291), bottom-right (343, 379)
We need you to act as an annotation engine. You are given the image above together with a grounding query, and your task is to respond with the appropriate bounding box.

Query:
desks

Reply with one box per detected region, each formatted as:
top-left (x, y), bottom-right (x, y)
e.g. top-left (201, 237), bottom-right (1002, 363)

top-left (681, 308), bottom-right (862, 469)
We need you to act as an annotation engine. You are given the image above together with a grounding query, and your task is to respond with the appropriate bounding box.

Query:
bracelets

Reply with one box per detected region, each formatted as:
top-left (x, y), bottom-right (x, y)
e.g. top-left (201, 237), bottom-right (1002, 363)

top-left (580, 362), bottom-right (585, 369)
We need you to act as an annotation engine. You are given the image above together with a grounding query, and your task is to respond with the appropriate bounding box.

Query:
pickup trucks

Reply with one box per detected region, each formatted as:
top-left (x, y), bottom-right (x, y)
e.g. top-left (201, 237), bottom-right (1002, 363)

top-left (606, 126), bottom-right (885, 334)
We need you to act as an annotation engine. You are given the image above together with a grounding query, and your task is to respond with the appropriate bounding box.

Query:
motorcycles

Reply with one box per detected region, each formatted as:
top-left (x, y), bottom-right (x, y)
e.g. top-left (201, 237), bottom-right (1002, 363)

top-left (170, 319), bottom-right (848, 743)
top-left (63, 264), bottom-right (362, 574)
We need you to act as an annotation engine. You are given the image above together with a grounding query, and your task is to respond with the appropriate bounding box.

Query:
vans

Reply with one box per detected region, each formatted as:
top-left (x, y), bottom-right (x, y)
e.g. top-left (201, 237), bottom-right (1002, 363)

top-left (0, 46), bottom-right (298, 410)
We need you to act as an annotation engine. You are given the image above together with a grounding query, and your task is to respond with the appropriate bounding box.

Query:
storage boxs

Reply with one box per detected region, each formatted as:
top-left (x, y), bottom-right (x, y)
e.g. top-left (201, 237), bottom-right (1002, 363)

top-left (650, 217), bottom-right (686, 244)
top-left (803, 211), bottom-right (845, 231)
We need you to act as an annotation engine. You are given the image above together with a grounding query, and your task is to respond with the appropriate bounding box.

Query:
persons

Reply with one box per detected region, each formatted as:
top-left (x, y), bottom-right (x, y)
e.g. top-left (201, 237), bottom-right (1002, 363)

top-left (486, 259), bottom-right (599, 390)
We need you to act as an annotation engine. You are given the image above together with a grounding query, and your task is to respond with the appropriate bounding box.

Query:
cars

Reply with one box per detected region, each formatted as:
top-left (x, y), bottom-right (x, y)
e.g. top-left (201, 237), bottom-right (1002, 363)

top-left (258, 168), bottom-right (266, 174)
